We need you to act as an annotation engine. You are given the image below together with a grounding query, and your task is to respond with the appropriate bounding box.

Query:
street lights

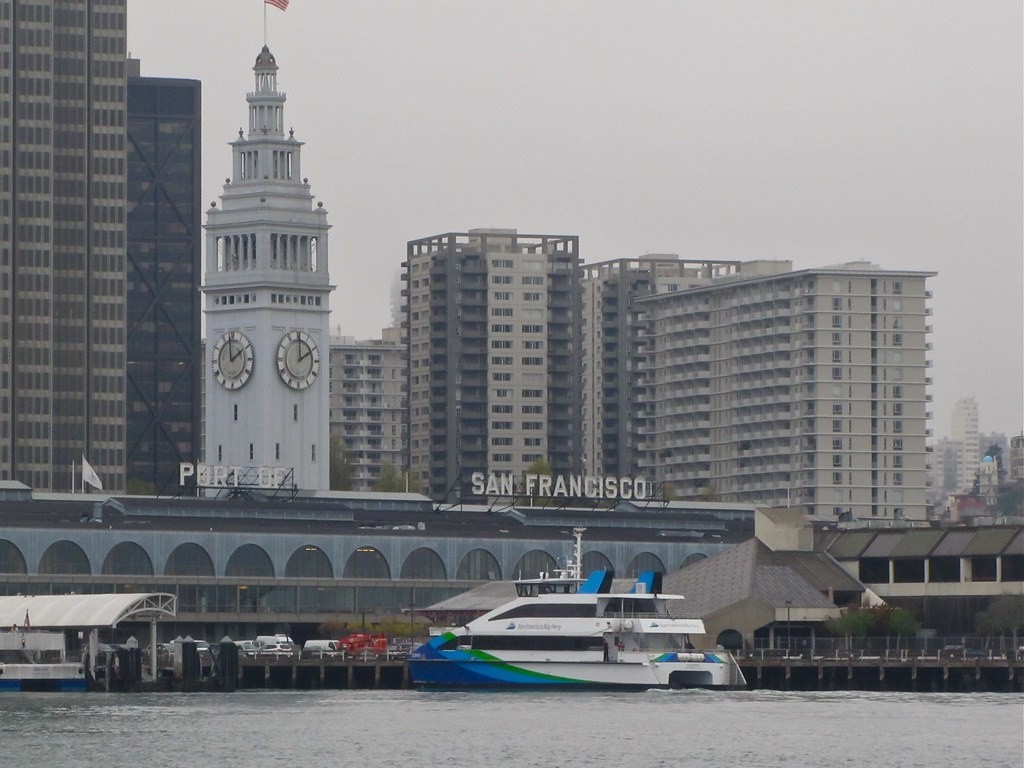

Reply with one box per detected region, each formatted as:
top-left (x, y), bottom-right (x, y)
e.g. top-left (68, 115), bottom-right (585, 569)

top-left (786, 599), bottom-right (793, 648)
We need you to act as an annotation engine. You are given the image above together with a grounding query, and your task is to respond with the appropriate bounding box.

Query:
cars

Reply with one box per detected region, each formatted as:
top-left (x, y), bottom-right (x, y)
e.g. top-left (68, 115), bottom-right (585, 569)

top-left (146, 635), bottom-right (423, 660)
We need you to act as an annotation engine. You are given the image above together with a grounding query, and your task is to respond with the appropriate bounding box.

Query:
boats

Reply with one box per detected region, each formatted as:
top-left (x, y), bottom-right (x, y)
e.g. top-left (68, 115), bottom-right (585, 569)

top-left (396, 524), bottom-right (748, 692)
